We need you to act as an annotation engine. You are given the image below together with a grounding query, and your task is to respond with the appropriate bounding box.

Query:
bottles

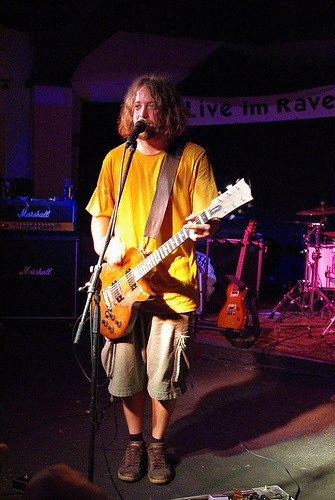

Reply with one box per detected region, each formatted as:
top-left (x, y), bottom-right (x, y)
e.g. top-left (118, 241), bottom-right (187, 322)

top-left (2, 176), bottom-right (11, 199)
top-left (63, 178), bottom-right (74, 200)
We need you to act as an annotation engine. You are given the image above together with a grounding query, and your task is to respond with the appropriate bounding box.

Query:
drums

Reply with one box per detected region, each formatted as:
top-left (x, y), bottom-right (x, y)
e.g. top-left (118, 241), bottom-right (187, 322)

top-left (305, 242), bottom-right (335, 292)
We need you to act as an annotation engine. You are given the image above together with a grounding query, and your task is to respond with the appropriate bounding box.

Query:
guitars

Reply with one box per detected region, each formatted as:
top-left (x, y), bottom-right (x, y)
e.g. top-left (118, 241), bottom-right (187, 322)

top-left (97, 177), bottom-right (257, 340)
top-left (217, 220), bottom-right (257, 331)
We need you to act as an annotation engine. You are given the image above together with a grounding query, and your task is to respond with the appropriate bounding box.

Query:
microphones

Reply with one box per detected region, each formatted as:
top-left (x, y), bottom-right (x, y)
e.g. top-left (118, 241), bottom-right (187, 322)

top-left (125, 119), bottom-right (147, 147)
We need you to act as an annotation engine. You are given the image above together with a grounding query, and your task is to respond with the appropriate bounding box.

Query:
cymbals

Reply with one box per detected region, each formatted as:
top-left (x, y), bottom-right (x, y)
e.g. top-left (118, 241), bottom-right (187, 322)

top-left (294, 207), bottom-right (335, 215)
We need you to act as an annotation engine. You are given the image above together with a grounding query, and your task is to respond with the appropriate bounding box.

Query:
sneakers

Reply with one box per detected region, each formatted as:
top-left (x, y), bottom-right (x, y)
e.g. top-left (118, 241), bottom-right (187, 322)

top-left (146, 442), bottom-right (171, 484)
top-left (118, 440), bottom-right (146, 482)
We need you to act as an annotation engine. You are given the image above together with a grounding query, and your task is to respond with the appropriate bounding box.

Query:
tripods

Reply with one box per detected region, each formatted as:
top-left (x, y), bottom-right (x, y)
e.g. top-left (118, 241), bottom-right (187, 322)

top-left (266, 213), bottom-right (335, 338)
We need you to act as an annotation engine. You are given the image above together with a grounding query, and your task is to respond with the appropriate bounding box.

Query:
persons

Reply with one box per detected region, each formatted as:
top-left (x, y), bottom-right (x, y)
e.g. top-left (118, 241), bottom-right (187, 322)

top-left (86, 74), bottom-right (221, 483)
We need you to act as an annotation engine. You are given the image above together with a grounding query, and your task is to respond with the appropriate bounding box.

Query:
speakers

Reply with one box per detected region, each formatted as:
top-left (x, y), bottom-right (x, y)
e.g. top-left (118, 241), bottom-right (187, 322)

top-left (0, 231), bottom-right (78, 321)
